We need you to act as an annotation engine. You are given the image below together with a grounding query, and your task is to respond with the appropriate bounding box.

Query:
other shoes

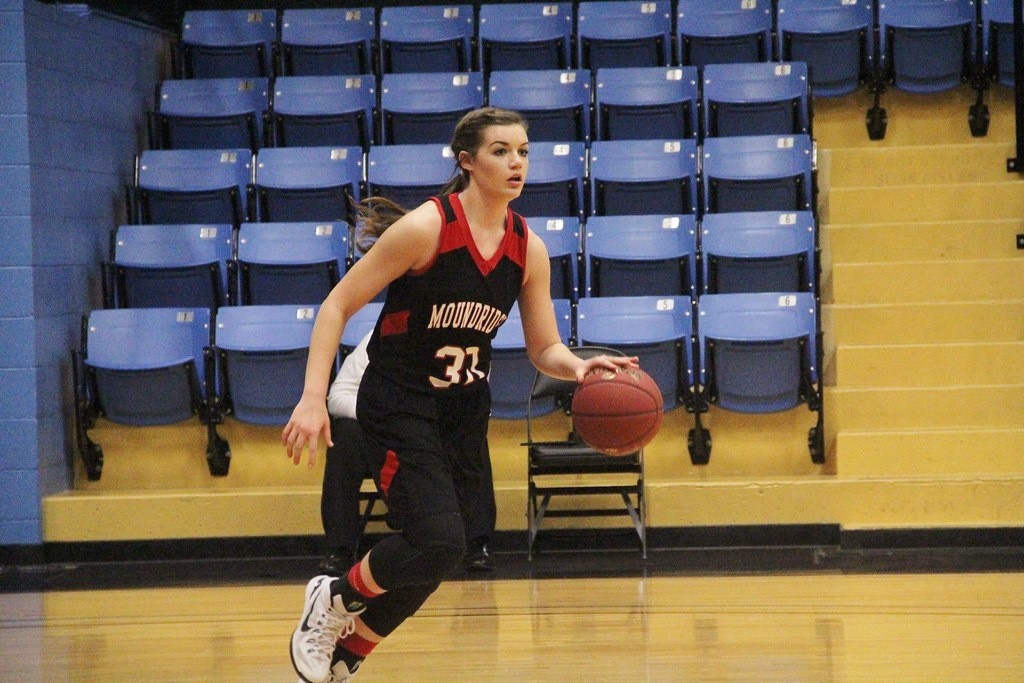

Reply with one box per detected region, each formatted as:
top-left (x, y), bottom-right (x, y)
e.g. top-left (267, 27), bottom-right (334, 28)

top-left (468, 544), bottom-right (495, 570)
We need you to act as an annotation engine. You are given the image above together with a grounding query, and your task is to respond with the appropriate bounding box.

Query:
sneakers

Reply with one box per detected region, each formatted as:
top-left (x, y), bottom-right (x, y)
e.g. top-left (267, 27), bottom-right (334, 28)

top-left (290, 574), bottom-right (367, 683)
top-left (299, 660), bottom-right (361, 683)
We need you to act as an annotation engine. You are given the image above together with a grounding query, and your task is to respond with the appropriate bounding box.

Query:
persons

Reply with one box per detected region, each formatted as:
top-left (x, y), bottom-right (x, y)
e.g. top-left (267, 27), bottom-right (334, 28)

top-left (319, 196), bottom-right (498, 578)
top-left (279, 106), bottom-right (639, 683)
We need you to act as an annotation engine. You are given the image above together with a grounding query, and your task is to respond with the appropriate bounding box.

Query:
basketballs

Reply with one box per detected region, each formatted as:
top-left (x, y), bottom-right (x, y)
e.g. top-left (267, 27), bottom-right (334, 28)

top-left (571, 366), bottom-right (663, 456)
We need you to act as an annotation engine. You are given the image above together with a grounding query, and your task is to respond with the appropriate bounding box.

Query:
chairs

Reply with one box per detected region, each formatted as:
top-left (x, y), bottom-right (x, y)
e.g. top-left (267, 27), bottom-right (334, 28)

top-left (68, 1), bottom-right (1023, 482)
top-left (519, 345), bottom-right (647, 570)
